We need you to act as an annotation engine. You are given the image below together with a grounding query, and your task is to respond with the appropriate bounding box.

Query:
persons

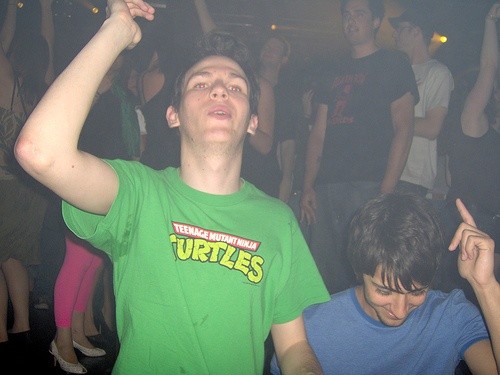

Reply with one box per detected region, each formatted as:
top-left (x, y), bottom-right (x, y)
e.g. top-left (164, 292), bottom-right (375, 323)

top-left (0, 0), bottom-right (334, 374)
top-left (269, 191), bottom-right (500, 375)
top-left (13, 0), bottom-right (332, 375)
top-left (389, 9), bottom-right (455, 197)
top-left (446, 1), bottom-right (499, 375)
top-left (298, 0), bottom-right (419, 296)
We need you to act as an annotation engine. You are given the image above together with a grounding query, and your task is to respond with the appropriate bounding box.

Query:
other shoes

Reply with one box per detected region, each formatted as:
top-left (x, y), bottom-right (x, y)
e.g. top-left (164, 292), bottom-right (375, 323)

top-left (86, 333), bottom-right (104, 344)
top-left (7, 329), bottom-right (32, 343)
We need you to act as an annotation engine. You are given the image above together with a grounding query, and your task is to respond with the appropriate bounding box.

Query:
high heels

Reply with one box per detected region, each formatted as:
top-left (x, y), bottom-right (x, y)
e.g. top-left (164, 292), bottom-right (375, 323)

top-left (71, 340), bottom-right (106, 356)
top-left (47, 340), bottom-right (87, 374)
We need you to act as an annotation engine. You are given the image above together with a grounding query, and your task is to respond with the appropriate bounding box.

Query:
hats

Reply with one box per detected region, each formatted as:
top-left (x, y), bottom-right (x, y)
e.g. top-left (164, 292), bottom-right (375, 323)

top-left (388, 9), bottom-right (433, 40)
